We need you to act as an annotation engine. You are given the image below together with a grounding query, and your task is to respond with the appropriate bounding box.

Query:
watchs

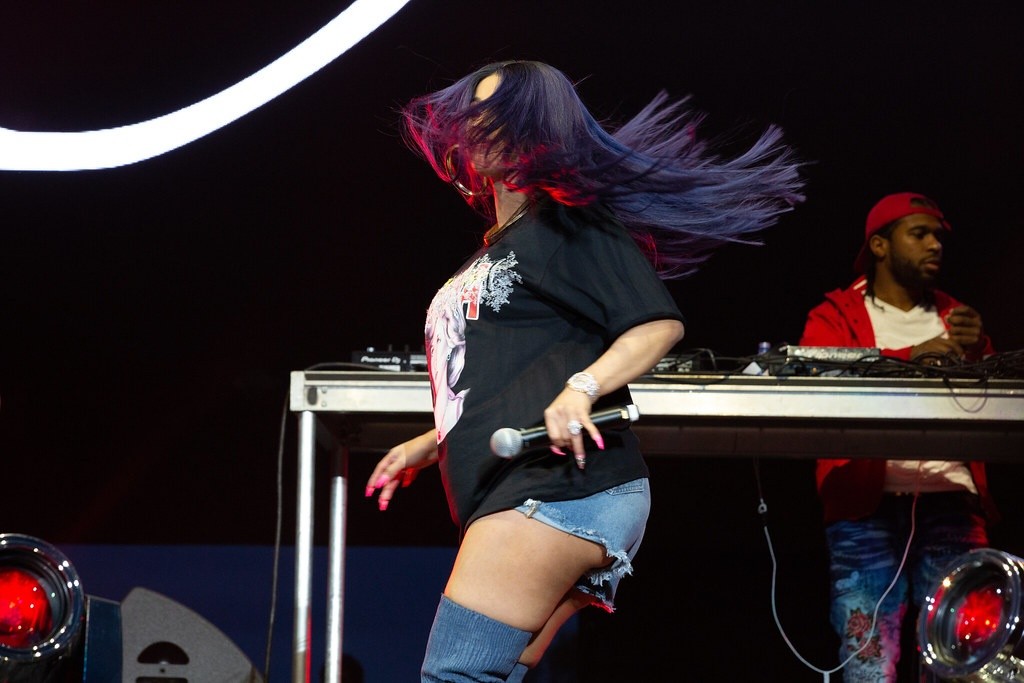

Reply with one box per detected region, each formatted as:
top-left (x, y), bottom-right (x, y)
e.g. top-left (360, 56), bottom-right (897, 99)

top-left (563, 370), bottom-right (602, 405)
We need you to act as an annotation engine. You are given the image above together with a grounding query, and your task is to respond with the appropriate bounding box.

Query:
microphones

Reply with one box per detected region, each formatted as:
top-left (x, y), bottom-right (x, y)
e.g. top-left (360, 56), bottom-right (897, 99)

top-left (491, 405), bottom-right (638, 456)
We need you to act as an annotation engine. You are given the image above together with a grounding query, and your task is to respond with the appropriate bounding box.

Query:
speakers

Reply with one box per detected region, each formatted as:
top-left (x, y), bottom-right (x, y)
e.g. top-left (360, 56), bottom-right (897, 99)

top-left (121, 587), bottom-right (262, 683)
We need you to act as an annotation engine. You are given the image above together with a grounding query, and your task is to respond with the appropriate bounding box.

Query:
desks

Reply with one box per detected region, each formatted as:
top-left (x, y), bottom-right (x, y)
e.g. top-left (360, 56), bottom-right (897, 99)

top-left (289, 345), bottom-right (1024, 683)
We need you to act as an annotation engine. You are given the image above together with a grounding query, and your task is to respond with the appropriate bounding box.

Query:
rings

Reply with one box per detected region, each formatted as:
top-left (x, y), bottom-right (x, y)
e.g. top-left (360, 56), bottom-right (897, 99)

top-left (567, 420), bottom-right (585, 435)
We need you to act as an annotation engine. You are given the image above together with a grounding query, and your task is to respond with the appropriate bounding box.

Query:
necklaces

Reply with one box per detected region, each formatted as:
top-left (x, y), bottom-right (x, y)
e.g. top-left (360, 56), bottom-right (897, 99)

top-left (481, 197), bottom-right (532, 247)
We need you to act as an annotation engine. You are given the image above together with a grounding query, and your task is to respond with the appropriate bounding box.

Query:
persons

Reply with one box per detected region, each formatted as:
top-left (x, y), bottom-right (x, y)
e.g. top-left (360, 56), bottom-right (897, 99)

top-left (365, 60), bottom-right (804, 683)
top-left (796, 192), bottom-right (1001, 683)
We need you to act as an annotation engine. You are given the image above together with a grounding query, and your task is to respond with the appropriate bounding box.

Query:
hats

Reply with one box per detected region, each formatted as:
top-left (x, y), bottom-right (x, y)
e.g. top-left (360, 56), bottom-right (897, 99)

top-left (851, 192), bottom-right (953, 274)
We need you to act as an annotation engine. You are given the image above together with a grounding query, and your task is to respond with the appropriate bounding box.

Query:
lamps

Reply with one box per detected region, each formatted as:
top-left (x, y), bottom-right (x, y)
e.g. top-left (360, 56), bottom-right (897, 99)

top-left (1, 532), bottom-right (86, 683)
top-left (916, 548), bottom-right (1024, 683)
